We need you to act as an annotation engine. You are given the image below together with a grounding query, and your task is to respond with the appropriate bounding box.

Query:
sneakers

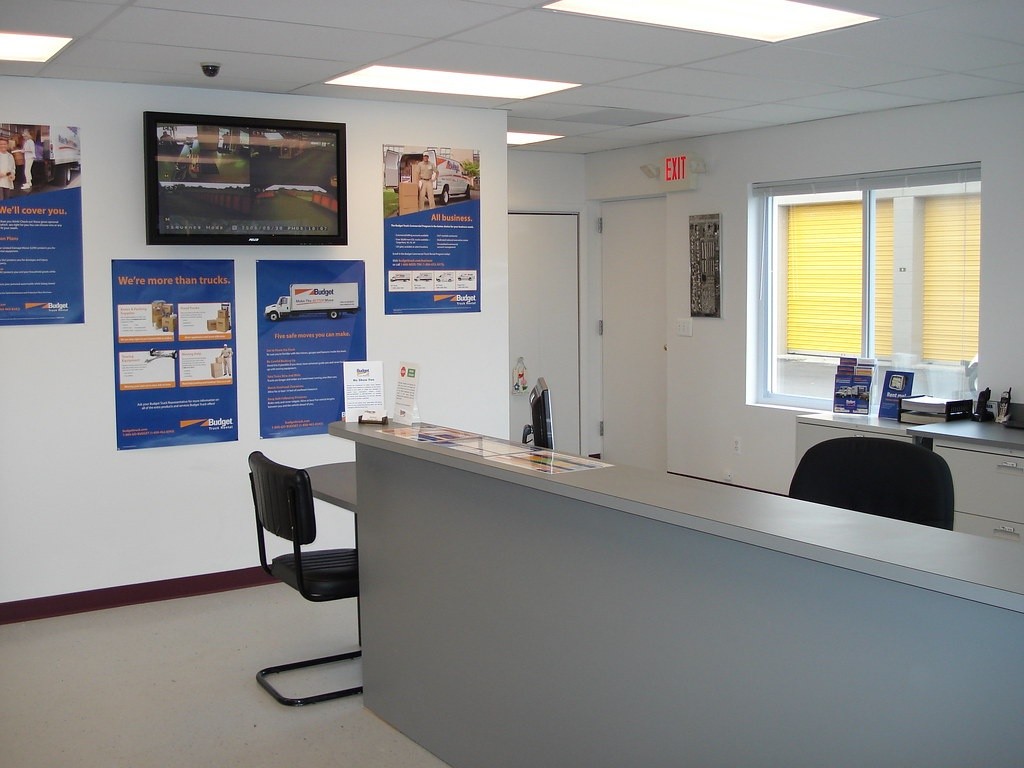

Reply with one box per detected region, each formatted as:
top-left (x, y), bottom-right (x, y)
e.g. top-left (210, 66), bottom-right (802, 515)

top-left (21, 183), bottom-right (32, 190)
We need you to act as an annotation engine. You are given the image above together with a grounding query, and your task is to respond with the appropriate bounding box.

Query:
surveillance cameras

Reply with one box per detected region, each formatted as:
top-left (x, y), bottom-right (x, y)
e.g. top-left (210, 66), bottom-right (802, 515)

top-left (199, 62), bottom-right (220, 77)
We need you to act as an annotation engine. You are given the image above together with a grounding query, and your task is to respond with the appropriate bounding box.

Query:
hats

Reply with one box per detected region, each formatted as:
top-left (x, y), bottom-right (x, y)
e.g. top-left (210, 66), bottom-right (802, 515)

top-left (423, 151), bottom-right (429, 157)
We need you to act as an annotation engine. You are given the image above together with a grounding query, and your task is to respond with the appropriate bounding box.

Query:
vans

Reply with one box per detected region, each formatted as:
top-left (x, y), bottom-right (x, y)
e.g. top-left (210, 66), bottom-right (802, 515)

top-left (384, 150), bottom-right (471, 204)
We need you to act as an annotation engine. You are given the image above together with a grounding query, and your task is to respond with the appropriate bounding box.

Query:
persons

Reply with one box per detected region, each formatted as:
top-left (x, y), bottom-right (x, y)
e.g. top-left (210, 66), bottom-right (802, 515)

top-left (0, 130), bottom-right (36, 200)
top-left (161, 132), bottom-right (173, 145)
top-left (222, 132), bottom-right (230, 152)
top-left (417, 152), bottom-right (439, 211)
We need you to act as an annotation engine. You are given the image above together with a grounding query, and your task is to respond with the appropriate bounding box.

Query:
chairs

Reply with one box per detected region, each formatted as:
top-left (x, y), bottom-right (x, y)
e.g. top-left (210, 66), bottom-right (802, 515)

top-left (785, 436), bottom-right (955, 532)
top-left (247, 450), bottom-right (362, 708)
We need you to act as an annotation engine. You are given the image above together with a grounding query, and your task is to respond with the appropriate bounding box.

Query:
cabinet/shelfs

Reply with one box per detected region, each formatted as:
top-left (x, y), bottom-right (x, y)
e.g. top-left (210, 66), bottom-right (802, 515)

top-left (906, 416), bottom-right (1024, 544)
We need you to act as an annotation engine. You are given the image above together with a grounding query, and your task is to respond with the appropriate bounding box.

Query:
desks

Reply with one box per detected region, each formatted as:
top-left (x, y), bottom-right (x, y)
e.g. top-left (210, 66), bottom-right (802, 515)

top-left (301, 460), bottom-right (370, 640)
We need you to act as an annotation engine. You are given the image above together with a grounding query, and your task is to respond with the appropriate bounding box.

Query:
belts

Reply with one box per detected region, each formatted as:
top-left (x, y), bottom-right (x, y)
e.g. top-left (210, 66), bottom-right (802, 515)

top-left (421, 178), bottom-right (431, 181)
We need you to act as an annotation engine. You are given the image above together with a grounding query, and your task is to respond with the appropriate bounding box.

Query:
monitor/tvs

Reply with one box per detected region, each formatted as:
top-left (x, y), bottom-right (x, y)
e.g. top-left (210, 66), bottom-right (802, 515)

top-left (142, 110), bottom-right (348, 245)
top-left (522, 377), bottom-right (554, 449)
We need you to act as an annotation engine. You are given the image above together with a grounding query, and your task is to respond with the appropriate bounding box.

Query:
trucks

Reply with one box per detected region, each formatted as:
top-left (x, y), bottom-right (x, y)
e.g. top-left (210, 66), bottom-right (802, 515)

top-left (265, 283), bottom-right (359, 322)
